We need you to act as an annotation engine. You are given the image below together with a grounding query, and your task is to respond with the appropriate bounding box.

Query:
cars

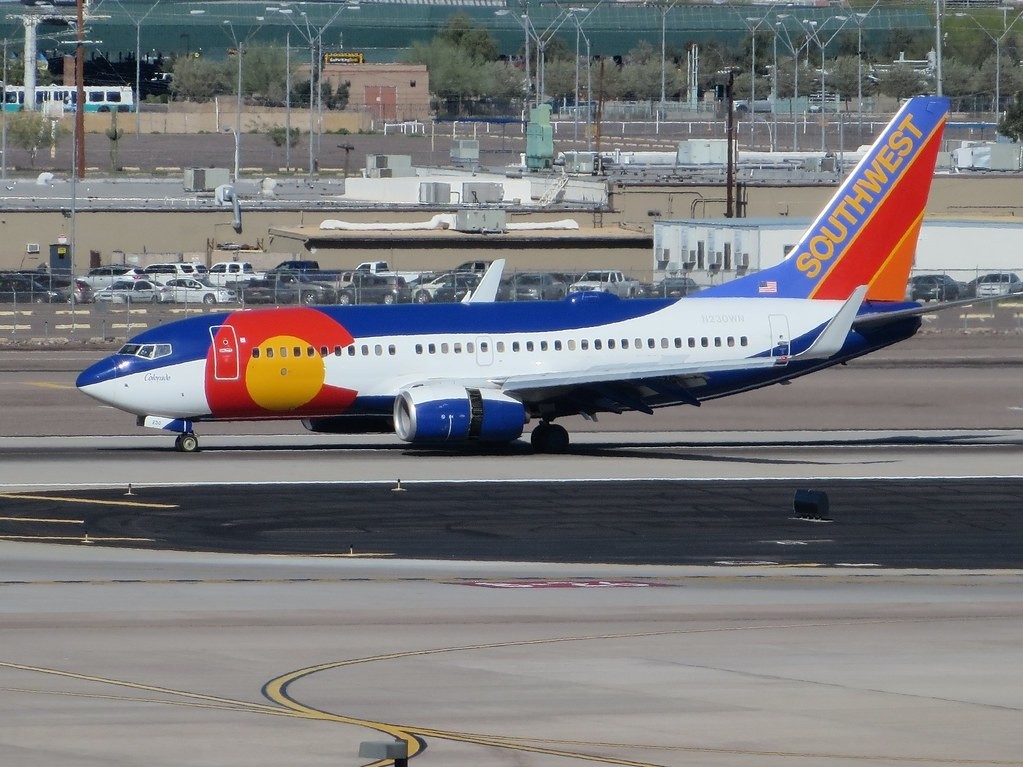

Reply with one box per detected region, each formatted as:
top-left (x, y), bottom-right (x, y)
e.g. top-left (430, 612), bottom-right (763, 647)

top-left (229, 259), bottom-right (515, 304)
top-left (955, 276), bottom-right (986, 300)
top-left (0, 269), bottom-right (94, 304)
top-left (163, 277), bottom-right (237, 305)
top-left (977, 273), bottom-right (1023, 295)
top-left (653, 278), bottom-right (700, 296)
top-left (911, 274), bottom-right (960, 302)
top-left (512, 273), bottom-right (569, 301)
top-left (94, 279), bottom-right (174, 304)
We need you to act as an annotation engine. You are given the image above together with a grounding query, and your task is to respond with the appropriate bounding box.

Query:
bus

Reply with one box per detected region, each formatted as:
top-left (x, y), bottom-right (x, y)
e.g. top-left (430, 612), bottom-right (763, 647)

top-left (0, 81), bottom-right (5, 111)
top-left (4, 84), bottom-right (132, 113)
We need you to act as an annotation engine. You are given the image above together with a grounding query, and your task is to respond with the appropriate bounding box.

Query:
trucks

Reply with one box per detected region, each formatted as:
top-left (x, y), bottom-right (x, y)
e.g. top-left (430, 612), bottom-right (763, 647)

top-left (355, 262), bottom-right (435, 283)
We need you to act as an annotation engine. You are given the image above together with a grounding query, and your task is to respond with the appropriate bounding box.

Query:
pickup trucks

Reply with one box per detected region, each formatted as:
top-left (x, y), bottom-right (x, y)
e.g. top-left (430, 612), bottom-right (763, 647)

top-left (208, 262), bottom-right (268, 282)
top-left (569, 269), bottom-right (641, 299)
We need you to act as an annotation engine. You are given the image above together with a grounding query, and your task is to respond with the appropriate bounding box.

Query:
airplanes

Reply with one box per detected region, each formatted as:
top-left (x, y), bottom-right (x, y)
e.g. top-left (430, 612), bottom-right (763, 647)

top-left (76, 94), bottom-right (1022, 462)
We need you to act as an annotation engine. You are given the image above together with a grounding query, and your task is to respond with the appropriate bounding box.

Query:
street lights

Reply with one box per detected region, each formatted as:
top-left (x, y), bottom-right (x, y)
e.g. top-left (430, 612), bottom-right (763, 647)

top-left (742, 12), bottom-right (870, 154)
top-left (491, 0), bottom-right (603, 151)
top-left (189, 0), bottom-right (362, 184)
top-left (955, 8), bottom-right (1022, 140)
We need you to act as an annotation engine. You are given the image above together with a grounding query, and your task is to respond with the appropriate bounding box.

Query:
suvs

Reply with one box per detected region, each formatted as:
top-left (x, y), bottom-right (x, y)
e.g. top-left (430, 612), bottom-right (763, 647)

top-left (75, 264), bottom-right (150, 291)
top-left (141, 261), bottom-right (210, 282)
